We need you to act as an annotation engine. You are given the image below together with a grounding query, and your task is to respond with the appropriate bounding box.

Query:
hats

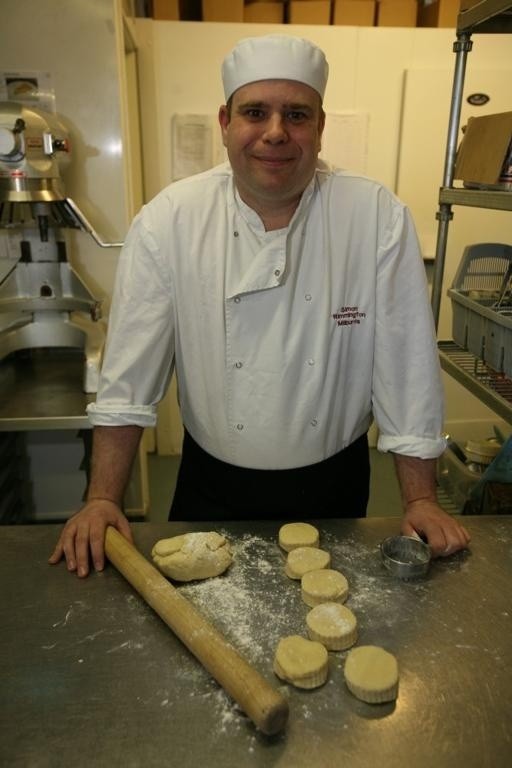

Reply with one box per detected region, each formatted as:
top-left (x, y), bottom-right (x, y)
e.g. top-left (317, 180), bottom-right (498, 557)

top-left (220, 34), bottom-right (330, 109)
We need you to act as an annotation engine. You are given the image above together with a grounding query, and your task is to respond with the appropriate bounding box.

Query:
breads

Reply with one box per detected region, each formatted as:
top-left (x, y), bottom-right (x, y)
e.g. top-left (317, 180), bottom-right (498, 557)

top-left (279, 522), bottom-right (320, 552)
top-left (273, 634), bottom-right (329, 690)
top-left (301, 569), bottom-right (349, 608)
top-left (286, 547), bottom-right (331, 580)
top-left (344, 645), bottom-right (399, 704)
top-left (306, 602), bottom-right (358, 652)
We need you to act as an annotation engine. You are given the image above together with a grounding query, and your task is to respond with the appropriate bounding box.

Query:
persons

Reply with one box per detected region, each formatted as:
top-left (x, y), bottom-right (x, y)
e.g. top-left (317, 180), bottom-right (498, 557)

top-left (48, 33), bottom-right (472, 578)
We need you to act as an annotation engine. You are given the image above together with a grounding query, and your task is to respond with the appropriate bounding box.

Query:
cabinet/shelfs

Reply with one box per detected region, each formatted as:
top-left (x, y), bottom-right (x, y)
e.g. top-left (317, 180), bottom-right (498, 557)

top-left (430, 0), bottom-right (512, 514)
